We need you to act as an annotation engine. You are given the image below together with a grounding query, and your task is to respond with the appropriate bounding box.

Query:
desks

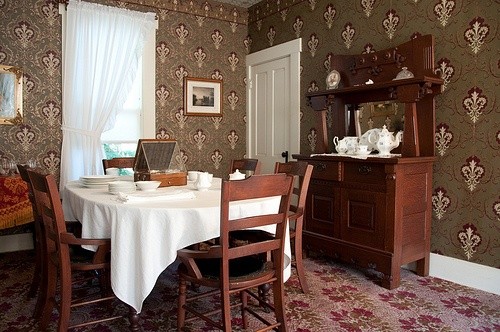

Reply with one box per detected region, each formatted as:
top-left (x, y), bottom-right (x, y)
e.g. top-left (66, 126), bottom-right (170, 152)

top-left (60, 180), bottom-right (292, 332)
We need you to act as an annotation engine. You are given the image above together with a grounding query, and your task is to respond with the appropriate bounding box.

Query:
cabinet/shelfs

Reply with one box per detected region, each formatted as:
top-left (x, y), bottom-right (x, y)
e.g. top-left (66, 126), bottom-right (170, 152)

top-left (291, 33), bottom-right (445, 291)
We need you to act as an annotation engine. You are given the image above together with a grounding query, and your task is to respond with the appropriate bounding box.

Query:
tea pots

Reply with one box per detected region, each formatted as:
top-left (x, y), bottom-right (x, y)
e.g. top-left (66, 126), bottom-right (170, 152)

top-left (369, 125), bottom-right (403, 154)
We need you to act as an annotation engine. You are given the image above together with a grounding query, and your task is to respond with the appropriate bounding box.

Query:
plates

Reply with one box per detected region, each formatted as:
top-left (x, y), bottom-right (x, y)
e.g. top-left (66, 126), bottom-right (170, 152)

top-left (326, 70), bottom-right (341, 87)
top-left (80, 175), bottom-right (135, 195)
top-left (355, 151), bottom-right (372, 155)
top-left (359, 129), bottom-right (380, 152)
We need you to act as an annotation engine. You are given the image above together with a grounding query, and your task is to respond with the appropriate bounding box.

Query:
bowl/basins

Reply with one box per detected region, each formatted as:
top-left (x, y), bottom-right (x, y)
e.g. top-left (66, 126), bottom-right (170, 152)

top-left (136, 181), bottom-right (163, 191)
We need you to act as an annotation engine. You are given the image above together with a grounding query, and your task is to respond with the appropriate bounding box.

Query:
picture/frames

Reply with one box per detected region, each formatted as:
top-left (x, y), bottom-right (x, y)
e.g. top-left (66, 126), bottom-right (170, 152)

top-left (182, 76), bottom-right (224, 117)
top-left (0, 64), bottom-right (24, 126)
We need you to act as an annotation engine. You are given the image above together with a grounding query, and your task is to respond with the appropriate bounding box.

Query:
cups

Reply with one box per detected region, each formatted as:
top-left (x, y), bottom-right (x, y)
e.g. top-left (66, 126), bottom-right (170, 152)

top-left (357, 145), bottom-right (368, 151)
top-left (106, 168), bottom-right (119, 175)
top-left (229, 170), bottom-right (246, 180)
top-left (188, 171), bottom-right (197, 180)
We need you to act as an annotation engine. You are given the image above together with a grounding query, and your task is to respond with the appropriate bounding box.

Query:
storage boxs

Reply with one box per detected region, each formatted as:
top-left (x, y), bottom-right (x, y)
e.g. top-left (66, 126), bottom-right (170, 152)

top-left (133, 138), bottom-right (188, 188)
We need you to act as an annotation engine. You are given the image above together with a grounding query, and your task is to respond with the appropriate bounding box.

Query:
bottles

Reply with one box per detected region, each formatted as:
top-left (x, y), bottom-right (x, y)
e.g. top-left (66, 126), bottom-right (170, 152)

top-left (194, 172), bottom-right (211, 191)
top-left (346, 136), bottom-right (359, 154)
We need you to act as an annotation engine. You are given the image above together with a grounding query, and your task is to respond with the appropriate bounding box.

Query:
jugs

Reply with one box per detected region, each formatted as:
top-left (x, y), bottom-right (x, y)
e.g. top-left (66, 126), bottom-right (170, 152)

top-left (333, 137), bottom-right (348, 154)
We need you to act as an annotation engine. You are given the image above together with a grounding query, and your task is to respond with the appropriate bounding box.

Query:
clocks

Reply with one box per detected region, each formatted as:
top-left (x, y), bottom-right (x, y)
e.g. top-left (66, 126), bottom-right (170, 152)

top-left (325, 70), bottom-right (340, 90)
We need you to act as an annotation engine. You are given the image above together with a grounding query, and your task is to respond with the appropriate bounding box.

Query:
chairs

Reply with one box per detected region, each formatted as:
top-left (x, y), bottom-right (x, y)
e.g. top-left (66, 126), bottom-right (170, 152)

top-left (176, 173), bottom-right (297, 332)
top-left (229, 157), bottom-right (259, 176)
top-left (15, 160), bottom-right (107, 318)
top-left (258, 162), bottom-right (316, 316)
top-left (25, 167), bottom-right (140, 332)
top-left (103, 156), bottom-right (136, 175)
